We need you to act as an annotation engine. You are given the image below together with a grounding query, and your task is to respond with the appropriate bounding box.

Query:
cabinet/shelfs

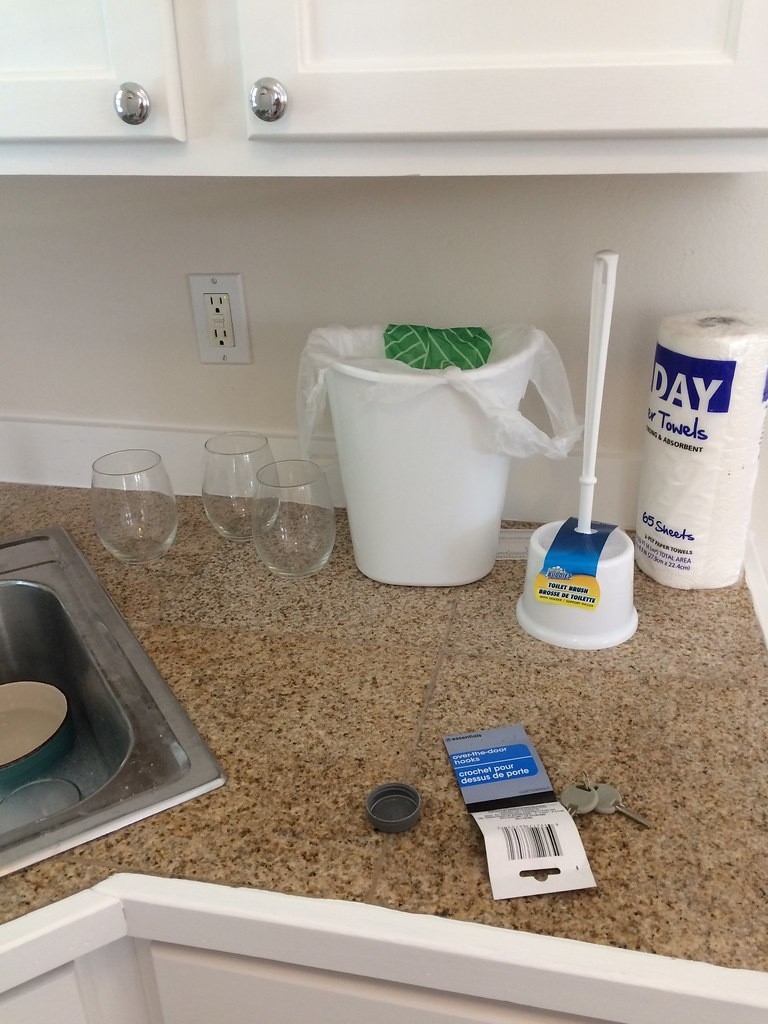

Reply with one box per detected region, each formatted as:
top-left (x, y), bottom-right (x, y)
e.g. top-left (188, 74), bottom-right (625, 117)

top-left (0, 0), bottom-right (768, 177)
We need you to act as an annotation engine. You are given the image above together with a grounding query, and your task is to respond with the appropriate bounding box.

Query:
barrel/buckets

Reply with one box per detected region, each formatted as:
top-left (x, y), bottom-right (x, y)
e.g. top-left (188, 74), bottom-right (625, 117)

top-left (306, 320), bottom-right (545, 590)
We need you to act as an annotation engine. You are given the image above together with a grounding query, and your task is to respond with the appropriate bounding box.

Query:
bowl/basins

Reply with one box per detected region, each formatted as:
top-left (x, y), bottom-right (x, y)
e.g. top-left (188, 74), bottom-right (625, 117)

top-left (0, 679), bottom-right (73, 796)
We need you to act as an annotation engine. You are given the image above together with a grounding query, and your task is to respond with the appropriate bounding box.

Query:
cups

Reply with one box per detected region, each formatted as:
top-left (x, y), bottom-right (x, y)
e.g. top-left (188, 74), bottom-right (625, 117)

top-left (202, 431), bottom-right (281, 541)
top-left (250, 458), bottom-right (337, 576)
top-left (0, 778), bottom-right (82, 830)
top-left (89, 449), bottom-right (178, 564)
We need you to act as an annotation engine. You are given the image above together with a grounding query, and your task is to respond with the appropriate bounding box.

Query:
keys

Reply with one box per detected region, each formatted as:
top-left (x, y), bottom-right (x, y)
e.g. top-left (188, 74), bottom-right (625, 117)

top-left (560, 769), bottom-right (654, 829)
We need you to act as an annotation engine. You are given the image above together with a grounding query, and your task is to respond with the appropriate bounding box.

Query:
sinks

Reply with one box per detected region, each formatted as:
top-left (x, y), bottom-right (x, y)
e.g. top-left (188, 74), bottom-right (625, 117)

top-left (1, 525), bottom-right (230, 885)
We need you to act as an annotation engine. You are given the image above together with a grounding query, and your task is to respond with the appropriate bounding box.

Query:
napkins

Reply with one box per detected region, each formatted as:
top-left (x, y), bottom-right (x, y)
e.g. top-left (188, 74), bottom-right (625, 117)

top-left (635, 310), bottom-right (768, 591)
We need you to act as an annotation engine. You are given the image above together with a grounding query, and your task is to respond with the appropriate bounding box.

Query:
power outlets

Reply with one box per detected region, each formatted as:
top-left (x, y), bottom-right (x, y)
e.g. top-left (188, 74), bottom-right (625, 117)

top-left (187, 273), bottom-right (250, 364)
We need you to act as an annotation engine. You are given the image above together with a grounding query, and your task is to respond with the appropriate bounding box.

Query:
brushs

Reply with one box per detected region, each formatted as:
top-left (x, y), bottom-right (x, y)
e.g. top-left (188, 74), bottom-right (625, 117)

top-left (518, 247), bottom-right (641, 653)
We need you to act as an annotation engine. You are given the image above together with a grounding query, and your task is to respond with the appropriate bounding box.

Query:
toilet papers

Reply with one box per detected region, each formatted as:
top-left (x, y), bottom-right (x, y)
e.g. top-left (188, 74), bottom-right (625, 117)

top-left (633, 306), bottom-right (768, 594)
